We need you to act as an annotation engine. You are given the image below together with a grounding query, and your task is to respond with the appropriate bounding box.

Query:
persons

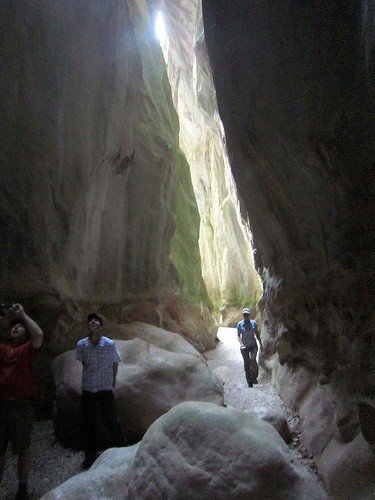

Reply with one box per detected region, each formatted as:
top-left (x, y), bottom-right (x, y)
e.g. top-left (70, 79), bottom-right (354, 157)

top-left (0, 301), bottom-right (43, 500)
top-left (237, 307), bottom-right (264, 390)
top-left (76, 312), bottom-right (121, 470)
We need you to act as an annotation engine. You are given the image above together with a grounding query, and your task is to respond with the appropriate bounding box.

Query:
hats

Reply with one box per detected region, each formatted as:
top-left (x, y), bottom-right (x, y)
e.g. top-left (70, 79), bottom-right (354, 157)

top-left (88, 313), bottom-right (103, 325)
top-left (242, 307), bottom-right (250, 315)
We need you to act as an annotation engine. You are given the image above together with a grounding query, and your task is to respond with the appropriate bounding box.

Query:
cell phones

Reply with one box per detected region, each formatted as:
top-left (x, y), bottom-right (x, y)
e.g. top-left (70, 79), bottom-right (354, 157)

top-left (1, 303), bottom-right (15, 311)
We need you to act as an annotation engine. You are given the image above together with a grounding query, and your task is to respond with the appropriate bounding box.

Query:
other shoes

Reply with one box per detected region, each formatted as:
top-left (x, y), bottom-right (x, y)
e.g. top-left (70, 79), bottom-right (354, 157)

top-left (252, 379), bottom-right (258, 384)
top-left (248, 383), bottom-right (253, 388)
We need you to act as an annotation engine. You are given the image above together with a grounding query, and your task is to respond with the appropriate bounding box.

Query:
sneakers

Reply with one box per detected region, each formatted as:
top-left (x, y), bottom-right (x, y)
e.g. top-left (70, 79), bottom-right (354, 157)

top-left (16, 486), bottom-right (27, 500)
top-left (81, 458), bottom-right (96, 469)
